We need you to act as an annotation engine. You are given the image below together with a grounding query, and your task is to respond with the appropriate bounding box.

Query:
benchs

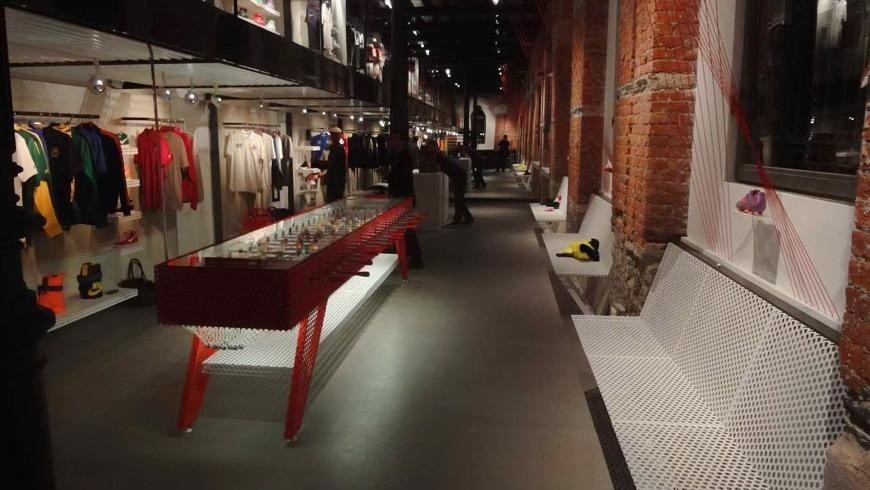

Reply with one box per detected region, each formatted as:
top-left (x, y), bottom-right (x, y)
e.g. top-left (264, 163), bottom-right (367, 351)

top-left (540, 194), bottom-right (613, 316)
top-left (570, 234), bottom-right (851, 488)
top-left (530, 175), bottom-right (570, 233)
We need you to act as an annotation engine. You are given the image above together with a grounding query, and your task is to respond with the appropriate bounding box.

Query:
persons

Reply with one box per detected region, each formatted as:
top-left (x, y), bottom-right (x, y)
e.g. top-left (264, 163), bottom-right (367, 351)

top-left (461, 146), bottom-right (487, 189)
top-left (495, 135), bottom-right (510, 172)
top-left (426, 154), bottom-right (473, 224)
top-left (318, 127), bottom-right (346, 203)
top-left (366, 38), bottom-right (385, 83)
top-left (377, 131), bottom-right (423, 270)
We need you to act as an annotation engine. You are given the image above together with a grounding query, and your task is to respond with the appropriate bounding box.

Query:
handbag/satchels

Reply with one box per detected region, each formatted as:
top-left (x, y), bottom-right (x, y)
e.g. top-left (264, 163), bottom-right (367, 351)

top-left (117, 258), bottom-right (158, 310)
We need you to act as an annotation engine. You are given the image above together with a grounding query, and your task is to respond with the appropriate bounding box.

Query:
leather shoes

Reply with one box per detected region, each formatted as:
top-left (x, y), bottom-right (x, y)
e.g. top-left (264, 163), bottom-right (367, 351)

top-left (405, 259), bottom-right (424, 270)
top-left (445, 215), bottom-right (475, 229)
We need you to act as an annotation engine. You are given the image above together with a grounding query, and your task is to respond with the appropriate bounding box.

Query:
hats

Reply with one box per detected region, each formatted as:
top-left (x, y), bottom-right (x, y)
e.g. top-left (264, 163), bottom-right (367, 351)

top-left (328, 127), bottom-right (342, 134)
